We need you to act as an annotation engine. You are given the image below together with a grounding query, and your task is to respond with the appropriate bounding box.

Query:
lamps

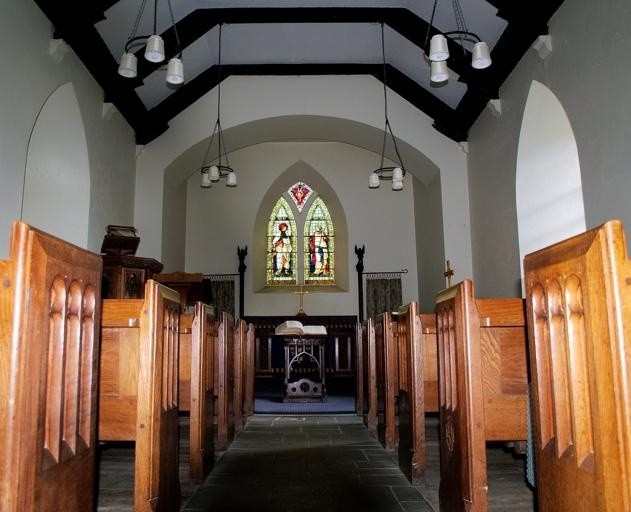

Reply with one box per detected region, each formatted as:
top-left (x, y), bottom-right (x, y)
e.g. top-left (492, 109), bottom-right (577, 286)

top-left (365, 16), bottom-right (410, 192)
top-left (117, 0), bottom-right (189, 88)
top-left (197, 19), bottom-right (242, 192)
top-left (424, 0), bottom-right (493, 83)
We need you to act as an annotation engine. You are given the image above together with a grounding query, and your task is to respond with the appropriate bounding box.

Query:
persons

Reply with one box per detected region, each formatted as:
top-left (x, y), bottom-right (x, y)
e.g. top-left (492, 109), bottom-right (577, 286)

top-left (271, 222), bottom-right (294, 277)
top-left (310, 224), bottom-right (333, 276)
